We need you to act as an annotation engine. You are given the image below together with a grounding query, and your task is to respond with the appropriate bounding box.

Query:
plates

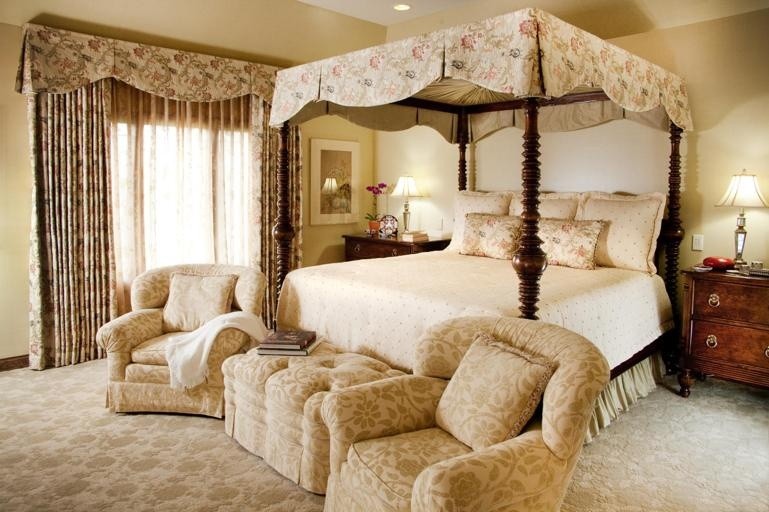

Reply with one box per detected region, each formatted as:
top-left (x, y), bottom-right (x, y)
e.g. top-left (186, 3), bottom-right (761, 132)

top-left (379, 215), bottom-right (398, 235)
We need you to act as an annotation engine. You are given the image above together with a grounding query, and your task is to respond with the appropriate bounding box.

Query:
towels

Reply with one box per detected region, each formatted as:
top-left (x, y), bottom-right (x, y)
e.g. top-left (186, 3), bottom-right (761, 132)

top-left (165, 310), bottom-right (268, 392)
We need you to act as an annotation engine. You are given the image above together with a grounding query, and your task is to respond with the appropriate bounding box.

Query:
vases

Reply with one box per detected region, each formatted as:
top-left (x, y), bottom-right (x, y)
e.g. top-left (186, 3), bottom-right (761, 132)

top-left (368, 219), bottom-right (381, 238)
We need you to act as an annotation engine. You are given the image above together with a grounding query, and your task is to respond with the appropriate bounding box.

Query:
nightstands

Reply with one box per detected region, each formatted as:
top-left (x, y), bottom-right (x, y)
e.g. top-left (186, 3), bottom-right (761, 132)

top-left (341, 232), bottom-right (451, 262)
top-left (677, 262), bottom-right (769, 398)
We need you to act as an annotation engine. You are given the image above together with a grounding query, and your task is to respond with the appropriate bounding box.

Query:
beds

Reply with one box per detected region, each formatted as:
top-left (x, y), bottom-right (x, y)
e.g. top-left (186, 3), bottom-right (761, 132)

top-left (266, 6), bottom-right (694, 446)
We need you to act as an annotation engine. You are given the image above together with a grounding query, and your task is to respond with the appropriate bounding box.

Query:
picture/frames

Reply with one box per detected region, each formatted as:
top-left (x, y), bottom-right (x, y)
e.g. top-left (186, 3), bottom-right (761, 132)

top-left (309, 137), bottom-right (362, 226)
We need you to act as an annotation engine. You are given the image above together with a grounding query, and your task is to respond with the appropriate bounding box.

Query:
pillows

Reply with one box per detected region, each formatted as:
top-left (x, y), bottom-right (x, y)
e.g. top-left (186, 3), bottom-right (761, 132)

top-left (508, 192), bottom-right (581, 222)
top-left (452, 189), bottom-right (514, 255)
top-left (536, 217), bottom-right (606, 269)
top-left (162, 272), bottom-right (240, 334)
top-left (435, 329), bottom-right (559, 452)
top-left (459, 212), bottom-right (522, 261)
top-left (575, 191), bottom-right (668, 277)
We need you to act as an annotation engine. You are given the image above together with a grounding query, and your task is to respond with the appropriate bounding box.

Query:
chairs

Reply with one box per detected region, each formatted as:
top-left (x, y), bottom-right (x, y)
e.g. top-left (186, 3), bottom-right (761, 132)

top-left (318, 316), bottom-right (609, 512)
top-left (96, 263), bottom-right (266, 420)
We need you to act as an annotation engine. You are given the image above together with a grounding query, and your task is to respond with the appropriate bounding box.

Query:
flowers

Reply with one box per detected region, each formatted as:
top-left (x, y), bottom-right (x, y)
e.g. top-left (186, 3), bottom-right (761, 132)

top-left (365, 182), bottom-right (389, 220)
top-left (340, 183), bottom-right (352, 213)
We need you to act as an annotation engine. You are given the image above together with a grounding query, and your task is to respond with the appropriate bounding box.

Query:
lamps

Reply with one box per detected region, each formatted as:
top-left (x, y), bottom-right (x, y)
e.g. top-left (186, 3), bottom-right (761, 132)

top-left (389, 176), bottom-right (424, 237)
top-left (712, 169), bottom-right (768, 272)
top-left (321, 177), bottom-right (339, 214)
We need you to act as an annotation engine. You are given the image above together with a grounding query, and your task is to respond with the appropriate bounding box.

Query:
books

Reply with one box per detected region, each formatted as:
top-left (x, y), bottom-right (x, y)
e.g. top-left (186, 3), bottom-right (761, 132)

top-left (257, 329), bottom-right (315, 350)
top-left (256, 332), bottom-right (326, 356)
top-left (400, 230), bottom-right (429, 242)
top-left (738, 265), bottom-right (768, 277)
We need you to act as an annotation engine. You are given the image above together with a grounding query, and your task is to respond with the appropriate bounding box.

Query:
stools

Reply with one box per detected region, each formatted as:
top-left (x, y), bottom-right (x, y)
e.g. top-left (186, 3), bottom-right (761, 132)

top-left (221, 340), bottom-right (410, 495)
